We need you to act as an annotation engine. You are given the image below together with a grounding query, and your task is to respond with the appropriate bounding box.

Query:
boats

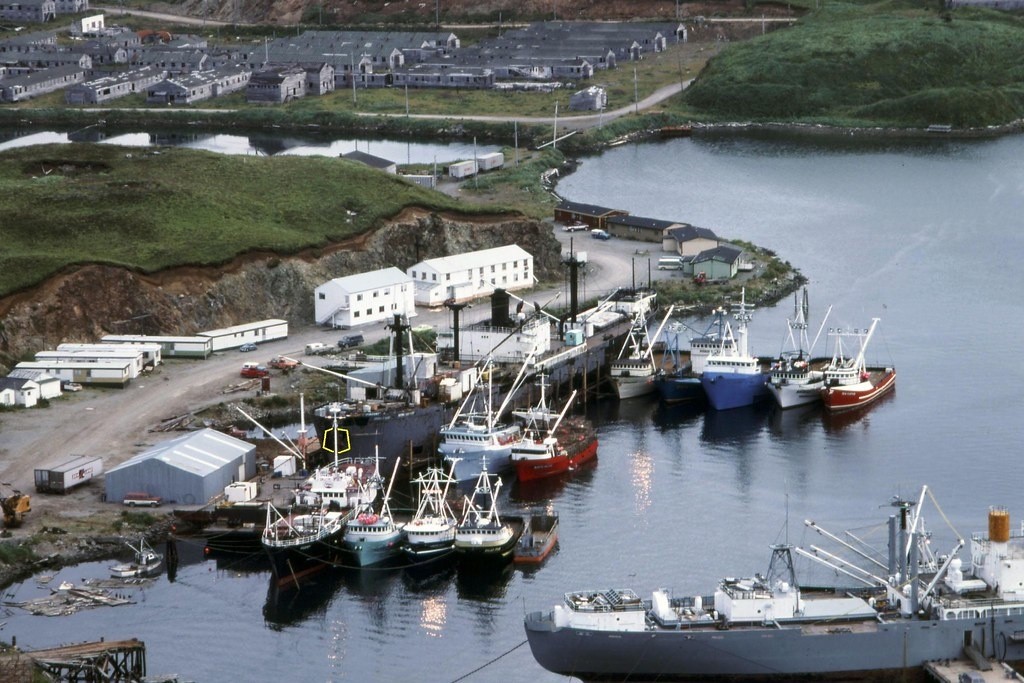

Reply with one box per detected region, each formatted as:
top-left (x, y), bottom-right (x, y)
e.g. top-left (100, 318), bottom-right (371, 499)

top-left (452, 454), bottom-right (523, 557)
top-left (763, 286), bottom-right (834, 410)
top-left (659, 125), bottom-right (693, 138)
top-left (260, 411), bottom-right (381, 549)
top-left (604, 287), bottom-right (691, 401)
top-left (263, 547), bottom-right (340, 620)
top-left (654, 321), bottom-right (705, 421)
top-left (107, 536), bottom-right (164, 579)
top-left (166, 393), bottom-right (325, 556)
top-left (313, 236), bottom-right (659, 465)
top-left (819, 317), bottom-right (896, 417)
top-left (524, 481), bottom-right (1023, 682)
top-left (513, 513), bottom-right (560, 566)
top-left (342, 443), bottom-right (403, 566)
top-left (699, 285), bottom-right (774, 410)
top-left (396, 456), bottom-right (465, 566)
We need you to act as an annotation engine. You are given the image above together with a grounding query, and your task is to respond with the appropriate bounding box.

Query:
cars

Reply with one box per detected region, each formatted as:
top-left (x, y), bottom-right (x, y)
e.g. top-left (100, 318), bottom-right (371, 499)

top-left (590, 227), bottom-right (610, 241)
top-left (269, 357), bottom-right (302, 372)
top-left (241, 360), bottom-right (269, 378)
top-left (239, 341), bottom-right (257, 353)
top-left (657, 251), bottom-right (686, 273)
top-left (339, 333), bottom-right (366, 350)
top-left (562, 220), bottom-right (589, 231)
top-left (306, 341), bottom-right (332, 357)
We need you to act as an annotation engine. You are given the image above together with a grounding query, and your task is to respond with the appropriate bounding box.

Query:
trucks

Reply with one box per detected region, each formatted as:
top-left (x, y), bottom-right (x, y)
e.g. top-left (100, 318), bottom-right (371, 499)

top-left (694, 271), bottom-right (729, 286)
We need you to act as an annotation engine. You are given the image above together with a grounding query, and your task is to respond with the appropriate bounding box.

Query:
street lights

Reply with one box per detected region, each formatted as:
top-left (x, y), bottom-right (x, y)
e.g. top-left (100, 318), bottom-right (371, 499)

top-left (474, 135), bottom-right (476, 192)
top-left (597, 86), bottom-right (602, 132)
top-left (351, 52), bottom-right (357, 104)
top-left (514, 119), bottom-right (518, 166)
top-left (435, 154), bottom-right (437, 185)
top-left (634, 67), bottom-right (638, 118)
top-left (405, 73), bottom-right (409, 118)
top-left (678, 53), bottom-right (684, 96)
top-left (553, 99), bottom-right (558, 148)
